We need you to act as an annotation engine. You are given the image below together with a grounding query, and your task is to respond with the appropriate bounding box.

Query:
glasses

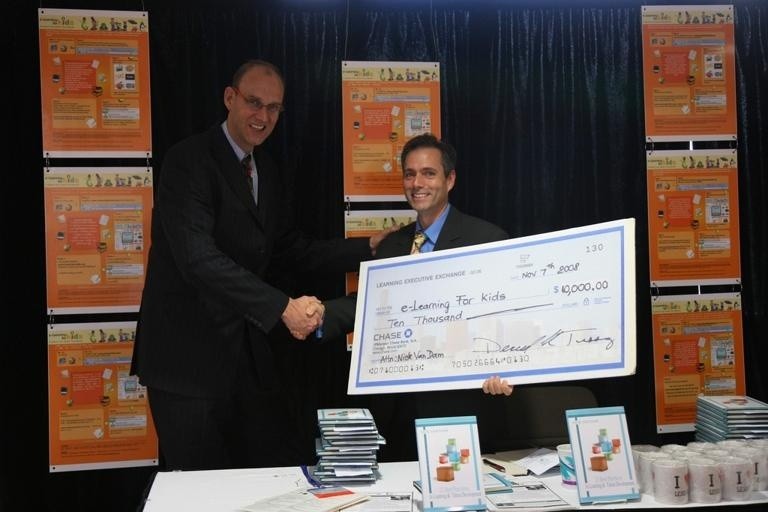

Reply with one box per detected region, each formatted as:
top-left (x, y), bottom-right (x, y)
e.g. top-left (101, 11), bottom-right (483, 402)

top-left (234, 88), bottom-right (284, 113)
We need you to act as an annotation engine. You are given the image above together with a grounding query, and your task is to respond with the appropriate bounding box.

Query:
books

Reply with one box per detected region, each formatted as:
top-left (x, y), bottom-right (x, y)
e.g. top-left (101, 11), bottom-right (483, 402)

top-left (240, 393), bottom-right (768, 510)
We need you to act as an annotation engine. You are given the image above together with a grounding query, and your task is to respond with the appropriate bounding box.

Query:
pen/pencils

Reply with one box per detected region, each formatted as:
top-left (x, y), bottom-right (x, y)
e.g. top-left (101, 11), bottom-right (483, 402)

top-left (390, 494), bottom-right (410, 500)
top-left (483, 458), bottom-right (506, 473)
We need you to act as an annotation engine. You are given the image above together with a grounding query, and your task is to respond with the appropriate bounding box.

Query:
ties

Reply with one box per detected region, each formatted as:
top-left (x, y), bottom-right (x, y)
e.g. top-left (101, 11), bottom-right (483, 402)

top-left (241, 154), bottom-right (254, 196)
top-left (411, 230), bottom-right (427, 255)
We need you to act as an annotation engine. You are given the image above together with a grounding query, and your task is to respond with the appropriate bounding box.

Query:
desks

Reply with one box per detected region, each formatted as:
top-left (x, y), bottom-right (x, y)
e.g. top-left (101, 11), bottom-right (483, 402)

top-left (139, 450), bottom-right (768, 511)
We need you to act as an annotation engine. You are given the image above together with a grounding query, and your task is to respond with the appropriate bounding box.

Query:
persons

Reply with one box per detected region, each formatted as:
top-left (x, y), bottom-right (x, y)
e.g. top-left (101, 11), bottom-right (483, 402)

top-left (127, 56), bottom-right (405, 474)
top-left (283, 133), bottom-right (515, 460)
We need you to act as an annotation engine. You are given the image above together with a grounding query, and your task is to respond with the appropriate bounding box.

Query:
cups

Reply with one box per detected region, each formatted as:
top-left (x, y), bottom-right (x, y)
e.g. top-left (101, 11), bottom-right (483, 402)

top-left (631, 438), bottom-right (768, 504)
top-left (557, 444), bottom-right (576, 486)
